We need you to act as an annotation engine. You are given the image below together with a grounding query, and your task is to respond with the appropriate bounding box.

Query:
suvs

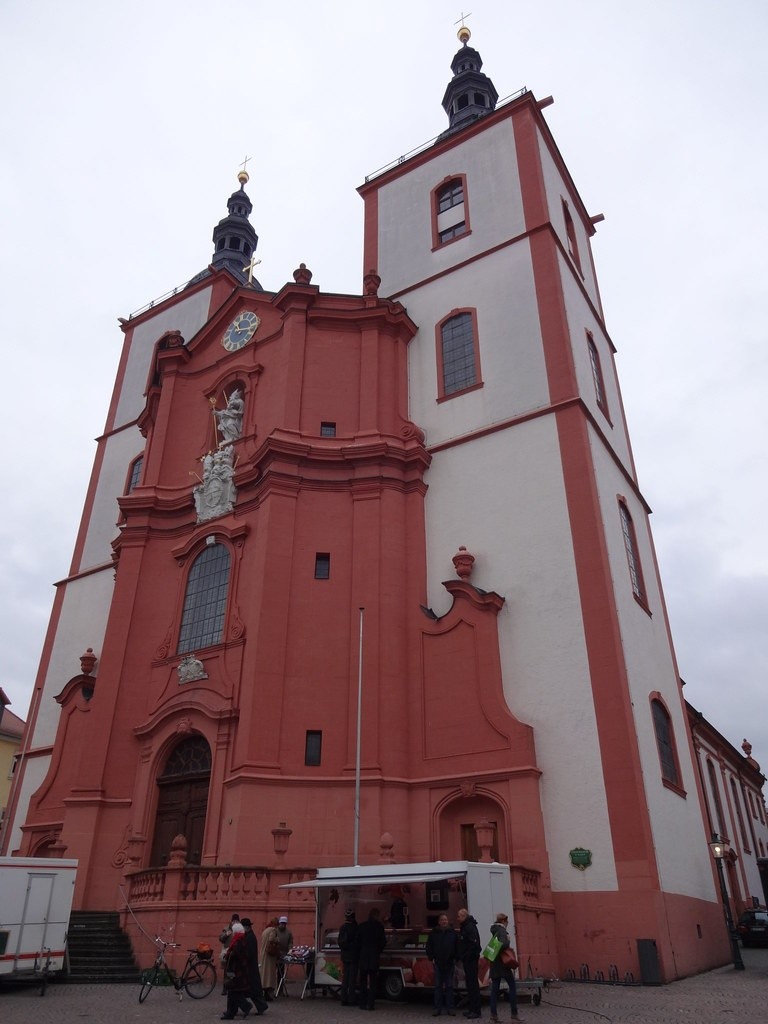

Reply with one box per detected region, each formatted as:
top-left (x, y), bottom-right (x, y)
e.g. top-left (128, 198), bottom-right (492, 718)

top-left (738, 907), bottom-right (768, 946)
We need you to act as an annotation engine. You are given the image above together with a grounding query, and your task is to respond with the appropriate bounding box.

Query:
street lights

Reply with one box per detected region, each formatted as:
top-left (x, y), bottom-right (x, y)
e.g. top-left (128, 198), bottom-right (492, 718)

top-left (707, 831), bottom-right (746, 970)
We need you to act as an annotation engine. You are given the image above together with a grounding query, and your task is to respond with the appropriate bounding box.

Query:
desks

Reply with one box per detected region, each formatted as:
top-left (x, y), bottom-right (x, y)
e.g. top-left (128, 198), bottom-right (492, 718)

top-left (273, 955), bottom-right (314, 1001)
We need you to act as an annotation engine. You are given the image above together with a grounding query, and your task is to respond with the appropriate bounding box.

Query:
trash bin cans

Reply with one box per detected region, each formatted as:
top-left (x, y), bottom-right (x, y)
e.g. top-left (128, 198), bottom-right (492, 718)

top-left (637, 938), bottom-right (661, 986)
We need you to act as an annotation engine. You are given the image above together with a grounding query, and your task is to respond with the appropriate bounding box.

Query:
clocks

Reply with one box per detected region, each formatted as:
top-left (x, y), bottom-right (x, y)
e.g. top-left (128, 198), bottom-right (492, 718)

top-left (222, 312), bottom-right (258, 351)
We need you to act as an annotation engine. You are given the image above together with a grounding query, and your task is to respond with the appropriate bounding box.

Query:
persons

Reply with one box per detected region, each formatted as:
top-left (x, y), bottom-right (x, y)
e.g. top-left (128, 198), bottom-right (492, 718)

top-left (356, 908), bottom-right (386, 1011)
top-left (490, 914), bottom-right (525, 1023)
top-left (426, 908), bottom-right (483, 1019)
top-left (391, 888), bottom-right (410, 928)
top-left (219, 913), bottom-right (293, 1019)
top-left (338, 908), bottom-right (358, 1006)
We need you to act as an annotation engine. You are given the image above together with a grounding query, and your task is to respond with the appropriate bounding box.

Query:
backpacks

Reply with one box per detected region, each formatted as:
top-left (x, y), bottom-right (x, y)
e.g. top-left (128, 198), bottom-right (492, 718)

top-left (338, 924), bottom-right (354, 947)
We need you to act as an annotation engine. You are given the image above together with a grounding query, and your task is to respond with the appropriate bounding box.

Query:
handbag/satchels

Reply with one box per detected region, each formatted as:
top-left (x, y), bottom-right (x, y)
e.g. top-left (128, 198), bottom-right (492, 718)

top-left (500, 947), bottom-right (520, 970)
top-left (223, 957), bottom-right (242, 989)
top-left (482, 932), bottom-right (503, 962)
top-left (265, 929), bottom-right (281, 957)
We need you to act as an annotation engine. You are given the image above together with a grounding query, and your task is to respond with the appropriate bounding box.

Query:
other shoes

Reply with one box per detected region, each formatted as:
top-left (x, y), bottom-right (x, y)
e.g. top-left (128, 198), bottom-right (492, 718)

top-left (221, 1012), bottom-right (237, 1019)
top-left (256, 1003), bottom-right (268, 1015)
top-left (341, 1000), bottom-right (358, 1006)
top-left (463, 1011), bottom-right (480, 1018)
top-left (432, 1009), bottom-right (441, 1016)
top-left (489, 1018), bottom-right (503, 1024)
top-left (448, 1009), bottom-right (456, 1016)
top-left (359, 1004), bottom-right (375, 1010)
top-left (511, 1017), bottom-right (525, 1022)
top-left (243, 1002), bottom-right (253, 1018)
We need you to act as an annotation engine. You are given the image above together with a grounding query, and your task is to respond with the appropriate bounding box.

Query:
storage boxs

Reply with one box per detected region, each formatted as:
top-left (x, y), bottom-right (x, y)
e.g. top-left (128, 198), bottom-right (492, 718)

top-left (142, 968), bottom-right (175, 986)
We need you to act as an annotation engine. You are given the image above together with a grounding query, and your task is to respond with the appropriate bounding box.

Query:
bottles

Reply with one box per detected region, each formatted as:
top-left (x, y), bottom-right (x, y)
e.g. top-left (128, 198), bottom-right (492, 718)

top-left (287, 945), bottom-right (315, 961)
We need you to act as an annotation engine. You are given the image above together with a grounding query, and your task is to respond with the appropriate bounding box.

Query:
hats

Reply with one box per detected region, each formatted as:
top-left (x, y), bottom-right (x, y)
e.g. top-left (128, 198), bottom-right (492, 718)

top-left (279, 916), bottom-right (288, 923)
top-left (232, 914), bottom-right (239, 921)
top-left (344, 908), bottom-right (355, 917)
top-left (240, 918), bottom-right (253, 925)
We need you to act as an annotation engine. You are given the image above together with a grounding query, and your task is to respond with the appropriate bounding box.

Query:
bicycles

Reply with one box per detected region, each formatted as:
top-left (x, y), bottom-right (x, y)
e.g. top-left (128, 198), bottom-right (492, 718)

top-left (138, 935), bottom-right (218, 1004)
